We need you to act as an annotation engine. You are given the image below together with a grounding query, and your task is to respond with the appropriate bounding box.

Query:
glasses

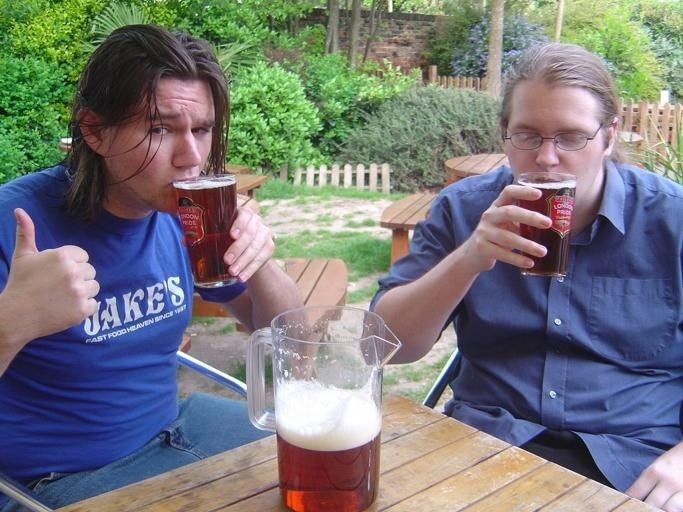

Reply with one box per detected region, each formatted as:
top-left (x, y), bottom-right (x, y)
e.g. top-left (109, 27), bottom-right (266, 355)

top-left (502, 121), bottom-right (603, 151)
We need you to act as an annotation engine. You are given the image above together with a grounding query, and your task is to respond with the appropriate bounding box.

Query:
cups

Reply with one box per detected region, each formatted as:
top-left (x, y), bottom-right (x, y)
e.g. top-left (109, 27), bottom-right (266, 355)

top-left (515, 170), bottom-right (579, 279)
top-left (172, 172), bottom-right (240, 291)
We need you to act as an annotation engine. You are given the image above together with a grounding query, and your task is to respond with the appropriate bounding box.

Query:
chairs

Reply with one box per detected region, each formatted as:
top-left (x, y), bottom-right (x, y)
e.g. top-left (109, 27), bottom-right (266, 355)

top-left (423, 346), bottom-right (460, 409)
top-left (0, 348), bottom-right (247, 512)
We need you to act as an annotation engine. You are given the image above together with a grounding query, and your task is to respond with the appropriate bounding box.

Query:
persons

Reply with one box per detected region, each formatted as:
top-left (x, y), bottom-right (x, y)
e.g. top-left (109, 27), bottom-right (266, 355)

top-left (360, 39), bottom-right (682, 512)
top-left (1, 22), bottom-right (312, 511)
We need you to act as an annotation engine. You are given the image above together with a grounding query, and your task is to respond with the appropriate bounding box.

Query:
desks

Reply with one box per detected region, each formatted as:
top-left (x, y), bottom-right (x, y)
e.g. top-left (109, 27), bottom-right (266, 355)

top-left (228, 173), bottom-right (266, 203)
top-left (442, 153), bottom-right (510, 192)
top-left (43, 393), bottom-right (672, 512)
top-left (57, 138), bottom-right (251, 175)
top-left (380, 191), bottom-right (437, 268)
top-left (192, 256), bottom-right (349, 384)
